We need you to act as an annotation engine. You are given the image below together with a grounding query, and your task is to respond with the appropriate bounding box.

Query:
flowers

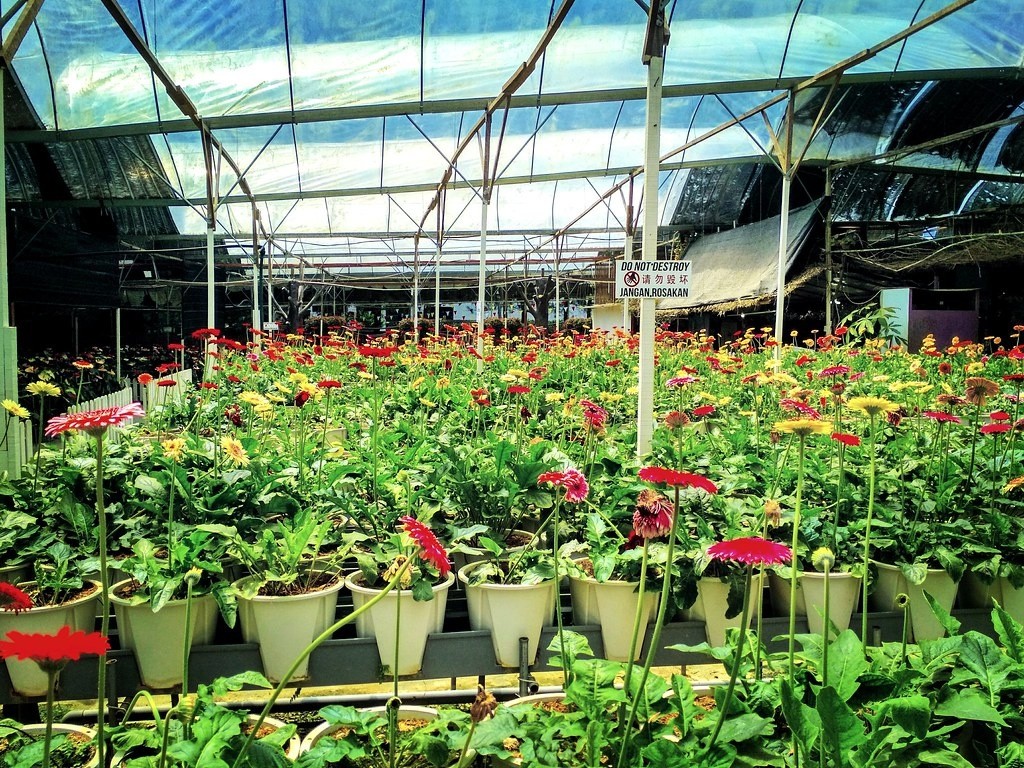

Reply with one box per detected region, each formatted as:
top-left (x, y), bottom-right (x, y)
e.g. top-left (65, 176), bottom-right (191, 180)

top-left (0, 304), bottom-right (1024, 768)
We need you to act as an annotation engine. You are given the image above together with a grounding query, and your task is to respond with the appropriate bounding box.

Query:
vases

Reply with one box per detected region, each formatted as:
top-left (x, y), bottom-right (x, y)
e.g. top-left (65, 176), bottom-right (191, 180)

top-left (344, 569), bottom-right (455, 676)
top-left (305, 554), bottom-right (343, 575)
top-left (0, 561), bottom-right (31, 582)
top-left (106, 577), bottom-right (217, 689)
top-left (452, 529), bottom-right (538, 572)
top-left (866, 559), bottom-right (964, 643)
top-left (650, 686), bottom-right (723, 746)
top-left (0, 579), bottom-right (104, 696)
top-left (109, 715), bottom-right (300, 768)
top-left (771, 569), bottom-right (863, 641)
top-left (312, 427), bottom-right (349, 446)
top-left (265, 511), bottom-right (349, 530)
top-left (566, 557), bottom-right (664, 661)
top-left (491, 692), bottom-right (570, 768)
top-left (272, 426), bottom-right (302, 441)
top-left (231, 569), bottom-right (345, 683)
top-left (970, 562), bottom-right (1024, 626)
top-left (299, 706), bottom-right (479, 768)
top-left (457, 560), bottom-right (565, 667)
top-left (680, 573), bottom-right (767, 647)
top-left (3, 724), bottom-right (107, 768)
